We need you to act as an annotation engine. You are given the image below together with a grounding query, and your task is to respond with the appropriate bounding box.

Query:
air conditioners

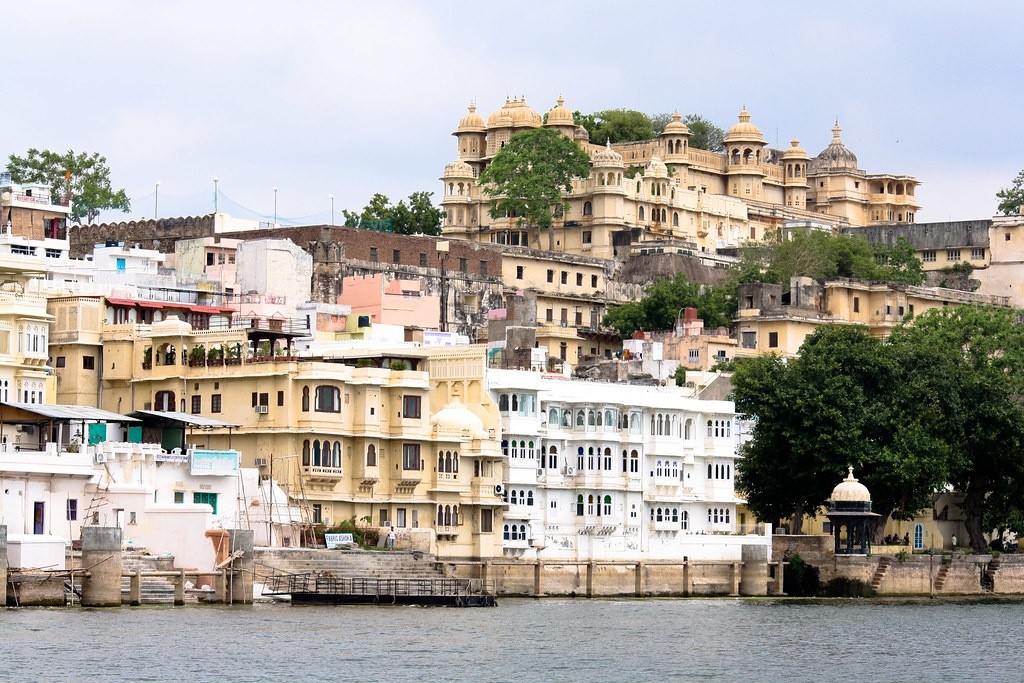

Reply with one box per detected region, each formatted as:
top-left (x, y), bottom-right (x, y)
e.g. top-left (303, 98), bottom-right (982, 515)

top-left (536, 468), bottom-right (545, 476)
top-left (256, 458), bottom-right (267, 466)
top-left (255, 405), bottom-right (267, 413)
top-left (565, 466), bottom-right (576, 476)
top-left (494, 484), bottom-right (505, 494)
top-left (95, 452), bottom-right (107, 463)
top-left (381, 521), bottom-right (391, 527)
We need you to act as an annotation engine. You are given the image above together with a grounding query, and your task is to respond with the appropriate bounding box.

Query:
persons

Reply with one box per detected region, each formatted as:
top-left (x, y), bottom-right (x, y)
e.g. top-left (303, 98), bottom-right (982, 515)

top-left (883, 533), bottom-right (902, 545)
top-left (388, 526), bottom-right (397, 551)
top-left (950, 534), bottom-right (957, 552)
top-left (903, 532), bottom-right (909, 545)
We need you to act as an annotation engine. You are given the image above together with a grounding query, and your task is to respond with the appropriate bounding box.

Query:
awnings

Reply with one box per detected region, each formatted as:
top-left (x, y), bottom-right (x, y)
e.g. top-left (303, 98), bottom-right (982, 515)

top-left (105, 295), bottom-right (239, 316)
top-left (5, 401), bottom-right (144, 446)
top-left (118, 405), bottom-right (242, 450)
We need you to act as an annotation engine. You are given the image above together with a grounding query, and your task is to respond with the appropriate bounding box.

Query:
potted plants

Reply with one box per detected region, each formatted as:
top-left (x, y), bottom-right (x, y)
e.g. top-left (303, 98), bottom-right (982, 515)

top-left (304, 525), bottom-right (379, 546)
